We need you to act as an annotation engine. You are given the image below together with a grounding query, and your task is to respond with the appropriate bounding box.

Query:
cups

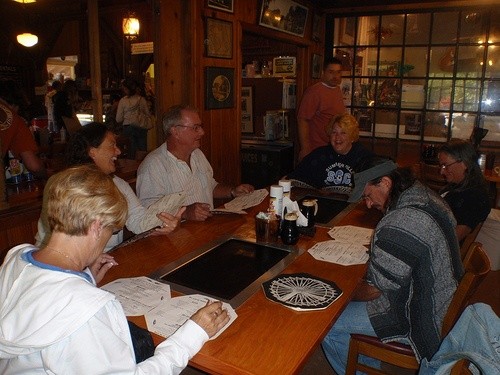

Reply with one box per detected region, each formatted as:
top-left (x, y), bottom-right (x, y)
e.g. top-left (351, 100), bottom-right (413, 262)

top-left (255, 213), bottom-right (281, 245)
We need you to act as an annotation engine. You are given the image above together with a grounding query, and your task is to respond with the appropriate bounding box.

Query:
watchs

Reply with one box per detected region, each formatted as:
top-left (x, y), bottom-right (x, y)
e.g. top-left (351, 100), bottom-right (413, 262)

top-left (231, 187), bottom-right (237, 198)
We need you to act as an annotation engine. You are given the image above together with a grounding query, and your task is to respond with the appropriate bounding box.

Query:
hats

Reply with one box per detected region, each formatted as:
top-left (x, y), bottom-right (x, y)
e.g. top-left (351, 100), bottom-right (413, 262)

top-left (347, 160), bottom-right (397, 203)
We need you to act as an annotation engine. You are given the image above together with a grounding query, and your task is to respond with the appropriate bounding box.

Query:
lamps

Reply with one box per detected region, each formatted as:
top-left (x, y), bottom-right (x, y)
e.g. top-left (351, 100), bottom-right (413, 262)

top-left (121, 6), bottom-right (141, 42)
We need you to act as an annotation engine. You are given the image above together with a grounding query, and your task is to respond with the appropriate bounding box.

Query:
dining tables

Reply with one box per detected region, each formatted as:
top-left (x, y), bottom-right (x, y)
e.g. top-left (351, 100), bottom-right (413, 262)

top-left (88, 180), bottom-right (382, 375)
top-left (0, 94), bottom-right (143, 265)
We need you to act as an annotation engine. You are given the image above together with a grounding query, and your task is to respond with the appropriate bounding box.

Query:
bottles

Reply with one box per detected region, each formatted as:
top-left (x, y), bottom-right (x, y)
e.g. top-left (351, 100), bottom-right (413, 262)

top-left (282, 213), bottom-right (298, 245)
top-left (301, 198), bottom-right (318, 234)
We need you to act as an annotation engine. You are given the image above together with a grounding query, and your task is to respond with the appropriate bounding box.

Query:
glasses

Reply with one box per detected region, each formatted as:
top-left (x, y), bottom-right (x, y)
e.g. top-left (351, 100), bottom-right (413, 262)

top-left (175, 124), bottom-right (204, 133)
top-left (327, 128), bottom-right (346, 135)
top-left (439, 160), bottom-right (462, 168)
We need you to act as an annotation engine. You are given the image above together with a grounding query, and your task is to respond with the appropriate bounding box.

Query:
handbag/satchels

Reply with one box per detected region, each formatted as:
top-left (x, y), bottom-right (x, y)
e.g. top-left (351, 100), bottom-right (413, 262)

top-left (132, 111), bottom-right (155, 129)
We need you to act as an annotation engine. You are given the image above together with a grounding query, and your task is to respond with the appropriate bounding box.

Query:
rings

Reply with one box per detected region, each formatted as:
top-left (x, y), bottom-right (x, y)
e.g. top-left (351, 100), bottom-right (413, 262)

top-left (213, 311), bottom-right (220, 318)
top-left (165, 224), bottom-right (169, 228)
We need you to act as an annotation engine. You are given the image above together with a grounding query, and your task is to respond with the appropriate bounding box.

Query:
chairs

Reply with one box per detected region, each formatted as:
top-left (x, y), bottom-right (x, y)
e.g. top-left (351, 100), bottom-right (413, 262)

top-left (346, 242), bottom-right (491, 374)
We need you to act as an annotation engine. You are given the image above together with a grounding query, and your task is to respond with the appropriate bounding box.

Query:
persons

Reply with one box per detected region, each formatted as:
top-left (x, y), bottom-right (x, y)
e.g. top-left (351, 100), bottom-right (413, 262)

top-left (318, 152), bottom-right (464, 375)
top-left (34, 121), bottom-right (187, 255)
top-left (135, 104), bottom-right (255, 222)
top-left (287, 112), bottom-right (379, 193)
top-left (115, 78), bottom-right (152, 158)
top-left (0, 80), bottom-right (61, 193)
top-left (434, 138), bottom-right (492, 247)
top-left (293, 57), bottom-right (348, 168)
top-left (45, 77), bottom-right (95, 143)
top-left (0, 165), bottom-right (231, 374)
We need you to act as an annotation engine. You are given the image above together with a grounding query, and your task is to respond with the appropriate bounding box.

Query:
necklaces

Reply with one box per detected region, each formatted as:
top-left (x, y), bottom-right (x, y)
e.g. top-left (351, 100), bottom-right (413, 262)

top-left (40, 246), bottom-right (82, 273)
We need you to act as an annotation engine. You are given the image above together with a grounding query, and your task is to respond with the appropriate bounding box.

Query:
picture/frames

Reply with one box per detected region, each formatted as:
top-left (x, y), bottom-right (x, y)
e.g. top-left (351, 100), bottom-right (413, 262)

top-left (204, 15), bottom-right (234, 60)
top-left (258, 0), bottom-right (310, 38)
top-left (205, 66), bottom-right (234, 109)
